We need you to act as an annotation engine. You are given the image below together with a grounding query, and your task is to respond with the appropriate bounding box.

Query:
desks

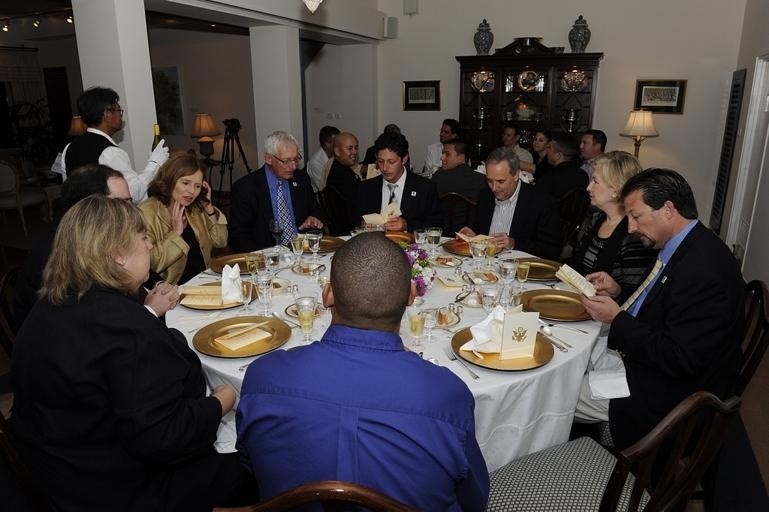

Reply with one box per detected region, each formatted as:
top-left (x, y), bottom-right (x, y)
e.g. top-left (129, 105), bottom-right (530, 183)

top-left (24, 180), bottom-right (65, 222)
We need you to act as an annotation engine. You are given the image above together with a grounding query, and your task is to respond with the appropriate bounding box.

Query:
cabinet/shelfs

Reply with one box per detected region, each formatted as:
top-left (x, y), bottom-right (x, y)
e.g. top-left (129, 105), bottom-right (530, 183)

top-left (553, 62), bottom-right (596, 134)
top-left (460, 63), bottom-right (500, 160)
top-left (502, 66), bottom-right (551, 147)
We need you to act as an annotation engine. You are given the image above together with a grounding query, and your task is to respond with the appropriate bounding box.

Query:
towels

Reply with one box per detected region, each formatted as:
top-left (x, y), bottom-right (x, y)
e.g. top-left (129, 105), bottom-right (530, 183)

top-left (589, 336), bottom-right (631, 400)
top-left (222, 263), bottom-right (242, 304)
top-left (459, 304), bottom-right (506, 355)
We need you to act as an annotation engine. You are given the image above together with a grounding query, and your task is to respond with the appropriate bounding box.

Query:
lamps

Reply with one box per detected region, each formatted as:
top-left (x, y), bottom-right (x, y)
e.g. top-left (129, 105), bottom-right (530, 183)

top-left (190, 113), bottom-right (226, 162)
top-left (67, 113), bottom-right (87, 138)
top-left (618, 106), bottom-right (659, 160)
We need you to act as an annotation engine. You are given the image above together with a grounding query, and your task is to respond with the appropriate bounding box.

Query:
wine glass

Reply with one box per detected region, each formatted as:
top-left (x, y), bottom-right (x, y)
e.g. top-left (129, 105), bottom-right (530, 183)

top-left (426, 227), bottom-right (442, 259)
top-left (484, 241), bottom-right (496, 272)
top-left (414, 228), bottom-right (427, 250)
top-left (269, 218), bottom-right (284, 244)
top-left (407, 309), bottom-right (426, 352)
top-left (252, 268), bottom-right (273, 318)
top-left (497, 257), bottom-right (517, 307)
top-left (469, 239), bottom-right (485, 273)
top-left (318, 305), bottom-right (334, 331)
top-left (294, 292), bottom-right (317, 345)
top-left (306, 231), bottom-right (323, 262)
top-left (481, 285), bottom-right (497, 315)
top-left (290, 237), bottom-right (304, 264)
top-left (516, 263), bottom-right (530, 291)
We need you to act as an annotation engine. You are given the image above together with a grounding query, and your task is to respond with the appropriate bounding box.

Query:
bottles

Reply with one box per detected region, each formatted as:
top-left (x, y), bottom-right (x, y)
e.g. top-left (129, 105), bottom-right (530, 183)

top-left (292, 285), bottom-right (298, 293)
top-left (309, 271), bottom-right (313, 278)
top-left (315, 270), bottom-right (321, 282)
top-left (472, 18), bottom-right (494, 56)
top-left (455, 269), bottom-right (459, 276)
top-left (568, 15), bottom-right (591, 53)
top-left (449, 303), bottom-right (454, 311)
top-left (458, 266), bottom-right (462, 274)
top-left (462, 285), bottom-right (467, 291)
top-left (151, 125), bottom-right (169, 169)
top-left (286, 286), bottom-right (290, 294)
top-left (456, 305), bottom-right (463, 320)
top-left (470, 283), bottom-right (475, 292)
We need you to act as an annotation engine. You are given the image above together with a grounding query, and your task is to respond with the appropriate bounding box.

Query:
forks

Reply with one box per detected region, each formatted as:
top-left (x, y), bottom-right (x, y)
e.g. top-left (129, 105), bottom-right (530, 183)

top-left (446, 349), bottom-right (478, 380)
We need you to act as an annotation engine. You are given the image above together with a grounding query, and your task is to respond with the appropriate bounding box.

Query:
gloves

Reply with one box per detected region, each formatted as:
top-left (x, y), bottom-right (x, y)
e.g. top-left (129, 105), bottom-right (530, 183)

top-left (148, 139), bottom-right (170, 167)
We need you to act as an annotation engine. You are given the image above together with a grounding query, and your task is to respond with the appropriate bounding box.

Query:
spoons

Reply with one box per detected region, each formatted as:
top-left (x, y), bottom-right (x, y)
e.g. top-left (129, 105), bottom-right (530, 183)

top-left (540, 325), bottom-right (573, 348)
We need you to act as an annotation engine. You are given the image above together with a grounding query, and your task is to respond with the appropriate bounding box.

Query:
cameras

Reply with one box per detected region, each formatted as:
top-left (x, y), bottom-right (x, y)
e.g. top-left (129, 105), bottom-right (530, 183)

top-left (222, 118), bottom-right (241, 130)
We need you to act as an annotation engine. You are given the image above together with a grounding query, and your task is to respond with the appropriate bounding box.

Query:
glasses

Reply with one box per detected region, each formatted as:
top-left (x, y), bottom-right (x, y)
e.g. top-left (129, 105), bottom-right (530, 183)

top-left (271, 152), bottom-right (303, 165)
top-left (107, 109), bottom-right (124, 118)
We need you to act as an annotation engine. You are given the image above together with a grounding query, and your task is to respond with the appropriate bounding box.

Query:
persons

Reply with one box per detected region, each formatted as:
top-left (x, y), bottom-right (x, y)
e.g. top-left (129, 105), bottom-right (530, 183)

top-left (489, 126), bottom-right (536, 173)
top-left (542, 138), bottom-right (590, 224)
top-left (325, 133), bottom-right (363, 237)
top-left (527, 131), bottom-right (548, 162)
top-left (228, 131), bottom-right (325, 254)
top-left (557, 151), bottom-right (660, 307)
top-left (423, 118), bottom-right (460, 174)
top-left (234, 232), bottom-right (489, 512)
top-left (355, 133), bottom-right (430, 234)
top-left (578, 128), bottom-right (607, 183)
top-left (306, 127), bottom-right (341, 213)
top-left (360, 124), bottom-right (411, 179)
top-left (7, 194), bottom-right (261, 511)
top-left (138, 154), bottom-right (229, 289)
top-left (60, 86), bottom-right (168, 202)
top-left (429, 138), bottom-right (489, 239)
top-left (14, 166), bottom-right (183, 333)
top-left (571, 168), bottom-right (744, 467)
top-left (456, 146), bottom-right (563, 260)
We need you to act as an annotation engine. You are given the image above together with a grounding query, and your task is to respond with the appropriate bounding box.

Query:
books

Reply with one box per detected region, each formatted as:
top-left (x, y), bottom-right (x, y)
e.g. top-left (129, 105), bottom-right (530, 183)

top-left (179, 284), bottom-right (224, 306)
top-left (213, 321), bottom-right (272, 351)
top-left (554, 264), bottom-right (597, 301)
top-left (499, 303), bottom-right (540, 361)
top-left (362, 201), bottom-right (403, 226)
top-left (453, 230), bottom-right (492, 244)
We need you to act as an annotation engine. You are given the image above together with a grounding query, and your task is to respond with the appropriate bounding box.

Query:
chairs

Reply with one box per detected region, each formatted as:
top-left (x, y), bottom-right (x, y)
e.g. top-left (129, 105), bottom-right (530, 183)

top-left (0, 159), bottom-right (50, 238)
top-left (596, 279), bottom-right (768, 511)
top-left (439, 193), bottom-right (479, 239)
top-left (487, 389), bottom-right (742, 511)
top-left (213, 479), bottom-right (416, 512)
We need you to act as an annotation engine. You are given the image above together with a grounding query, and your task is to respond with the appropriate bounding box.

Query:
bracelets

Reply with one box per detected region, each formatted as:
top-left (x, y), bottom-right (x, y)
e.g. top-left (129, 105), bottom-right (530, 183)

top-left (207, 211), bottom-right (217, 217)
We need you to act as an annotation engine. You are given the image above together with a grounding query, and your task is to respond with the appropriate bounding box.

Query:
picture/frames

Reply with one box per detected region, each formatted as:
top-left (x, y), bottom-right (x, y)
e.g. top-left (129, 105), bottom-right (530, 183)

top-left (403, 80), bottom-right (440, 112)
top-left (635, 79), bottom-right (686, 113)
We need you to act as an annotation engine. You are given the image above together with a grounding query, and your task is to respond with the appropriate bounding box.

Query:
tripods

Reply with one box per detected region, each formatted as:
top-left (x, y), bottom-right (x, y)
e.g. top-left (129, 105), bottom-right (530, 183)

top-left (217, 131), bottom-right (250, 202)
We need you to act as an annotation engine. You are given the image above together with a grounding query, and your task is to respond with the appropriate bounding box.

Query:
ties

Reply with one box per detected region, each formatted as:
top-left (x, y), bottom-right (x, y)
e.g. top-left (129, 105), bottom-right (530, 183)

top-left (621, 258), bottom-right (664, 311)
top-left (388, 184), bottom-right (397, 205)
top-left (276, 180), bottom-right (297, 247)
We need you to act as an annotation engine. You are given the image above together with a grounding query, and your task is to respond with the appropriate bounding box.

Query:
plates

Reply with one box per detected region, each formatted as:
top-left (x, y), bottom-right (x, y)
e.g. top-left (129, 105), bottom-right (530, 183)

top-left (191, 316), bottom-right (292, 360)
top-left (291, 263), bottom-right (326, 277)
top-left (451, 322), bottom-right (554, 372)
top-left (270, 278), bottom-right (290, 292)
top-left (456, 291), bottom-right (498, 309)
top-left (462, 271), bottom-right (498, 287)
top-left (429, 256), bottom-right (463, 269)
top-left (414, 308), bottom-right (460, 328)
top-left (517, 288), bottom-right (597, 322)
top-left (179, 279), bottom-right (261, 311)
top-left (498, 257), bottom-right (567, 282)
top-left (287, 235), bottom-right (348, 253)
top-left (285, 302), bottom-right (327, 318)
top-left (210, 252), bottom-right (267, 275)
top-left (442, 235), bottom-right (504, 258)
top-left (384, 230), bottom-right (415, 249)
